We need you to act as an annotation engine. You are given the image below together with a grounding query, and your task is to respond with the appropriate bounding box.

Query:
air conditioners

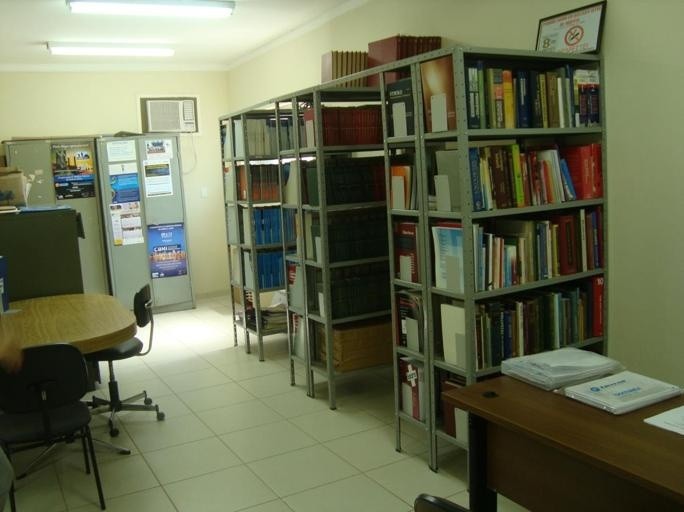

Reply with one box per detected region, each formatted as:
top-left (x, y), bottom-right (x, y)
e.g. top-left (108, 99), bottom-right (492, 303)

top-left (141, 98), bottom-right (197, 136)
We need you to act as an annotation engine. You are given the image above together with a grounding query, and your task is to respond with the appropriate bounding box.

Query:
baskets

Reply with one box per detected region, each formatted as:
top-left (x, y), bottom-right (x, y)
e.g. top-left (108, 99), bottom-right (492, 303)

top-left (317, 322), bottom-right (393, 373)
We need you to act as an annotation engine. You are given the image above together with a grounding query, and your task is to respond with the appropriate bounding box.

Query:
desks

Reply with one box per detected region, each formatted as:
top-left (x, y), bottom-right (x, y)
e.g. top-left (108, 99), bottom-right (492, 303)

top-left (412, 377), bottom-right (684, 511)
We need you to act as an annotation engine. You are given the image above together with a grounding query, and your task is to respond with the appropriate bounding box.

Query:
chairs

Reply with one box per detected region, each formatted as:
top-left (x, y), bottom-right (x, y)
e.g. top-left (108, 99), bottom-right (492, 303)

top-left (2, 283), bottom-right (165, 510)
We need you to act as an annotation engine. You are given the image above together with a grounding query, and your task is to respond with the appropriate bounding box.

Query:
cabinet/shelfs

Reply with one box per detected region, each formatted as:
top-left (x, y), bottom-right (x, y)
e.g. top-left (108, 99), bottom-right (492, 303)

top-left (1, 133), bottom-right (198, 393)
top-left (214, 39), bottom-right (611, 476)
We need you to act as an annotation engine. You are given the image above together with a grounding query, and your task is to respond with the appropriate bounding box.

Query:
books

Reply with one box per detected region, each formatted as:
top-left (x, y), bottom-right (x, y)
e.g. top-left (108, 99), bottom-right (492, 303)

top-left (565, 370), bottom-right (683, 417)
top-left (384, 53), bottom-right (603, 443)
top-left (321, 35), bottom-right (442, 88)
top-left (222, 119), bottom-right (293, 331)
top-left (281, 103), bottom-right (389, 369)
